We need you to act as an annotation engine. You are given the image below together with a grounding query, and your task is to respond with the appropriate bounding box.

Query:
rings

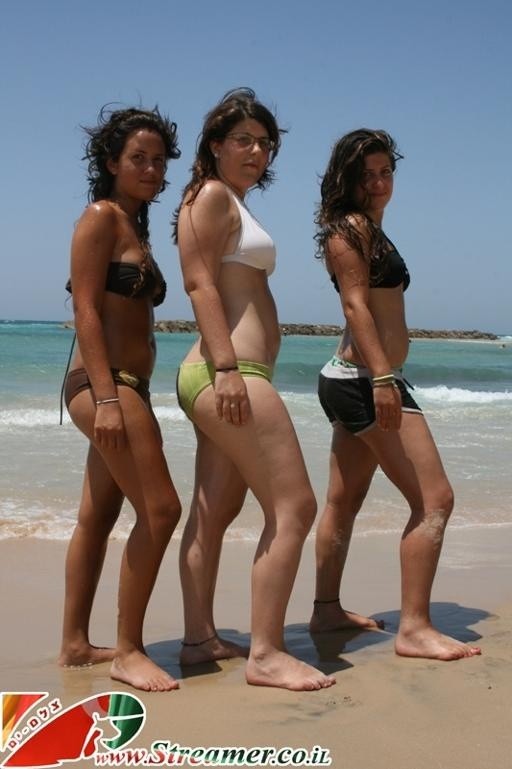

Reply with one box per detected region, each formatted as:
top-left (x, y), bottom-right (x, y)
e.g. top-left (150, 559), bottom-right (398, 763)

top-left (229, 402), bottom-right (240, 409)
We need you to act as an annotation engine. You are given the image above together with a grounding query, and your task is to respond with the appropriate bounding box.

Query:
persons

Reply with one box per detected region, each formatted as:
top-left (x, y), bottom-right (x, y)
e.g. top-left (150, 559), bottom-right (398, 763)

top-left (58, 101), bottom-right (185, 694)
top-left (171, 83), bottom-right (339, 693)
top-left (307, 125), bottom-right (486, 665)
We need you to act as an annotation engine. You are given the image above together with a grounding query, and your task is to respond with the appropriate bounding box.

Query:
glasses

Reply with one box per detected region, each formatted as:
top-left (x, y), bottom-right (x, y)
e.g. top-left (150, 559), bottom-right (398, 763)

top-left (222, 134), bottom-right (270, 150)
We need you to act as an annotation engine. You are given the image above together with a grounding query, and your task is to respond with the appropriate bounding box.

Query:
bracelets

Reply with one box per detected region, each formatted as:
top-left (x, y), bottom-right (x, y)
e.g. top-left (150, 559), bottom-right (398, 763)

top-left (372, 378), bottom-right (395, 388)
top-left (93, 398), bottom-right (122, 407)
top-left (370, 373), bottom-right (397, 382)
top-left (215, 366), bottom-right (243, 373)
